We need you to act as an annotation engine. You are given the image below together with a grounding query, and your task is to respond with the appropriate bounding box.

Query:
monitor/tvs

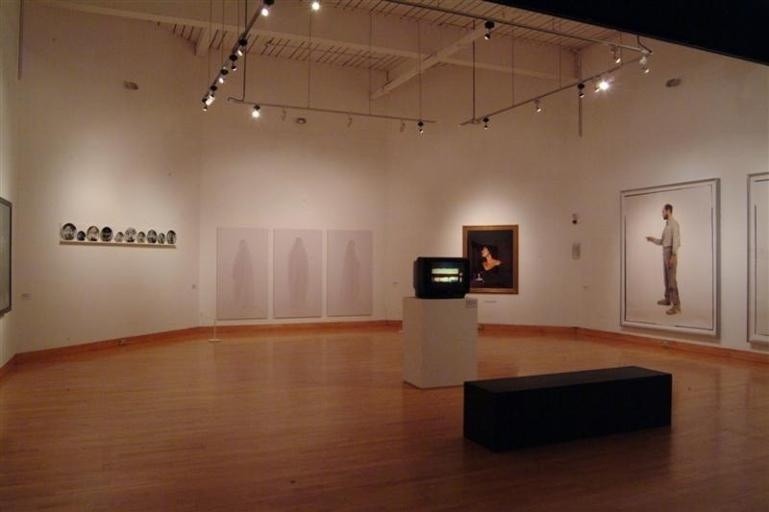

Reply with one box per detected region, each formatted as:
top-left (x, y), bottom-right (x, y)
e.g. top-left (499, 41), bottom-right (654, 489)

top-left (413, 257), bottom-right (470, 299)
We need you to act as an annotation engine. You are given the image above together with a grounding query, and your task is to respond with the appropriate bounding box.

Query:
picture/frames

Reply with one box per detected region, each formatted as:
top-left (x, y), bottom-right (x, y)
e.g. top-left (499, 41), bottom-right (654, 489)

top-left (748, 172), bottom-right (769, 344)
top-left (0, 196), bottom-right (12, 315)
top-left (463, 225), bottom-right (519, 295)
top-left (618, 179), bottom-right (722, 339)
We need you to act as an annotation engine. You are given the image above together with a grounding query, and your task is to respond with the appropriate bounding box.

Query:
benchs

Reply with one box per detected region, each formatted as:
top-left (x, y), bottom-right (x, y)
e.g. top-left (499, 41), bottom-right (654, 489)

top-left (464, 366), bottom-right (672, 453)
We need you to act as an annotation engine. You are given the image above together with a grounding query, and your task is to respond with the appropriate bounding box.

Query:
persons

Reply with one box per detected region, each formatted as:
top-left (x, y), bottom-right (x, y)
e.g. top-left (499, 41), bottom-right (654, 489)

top-left (60, 222), bottom-right (177, 244)
top-left (643, 203), bottom-right (683, 316)
top-left (477, 244), bottom-right (506, 284)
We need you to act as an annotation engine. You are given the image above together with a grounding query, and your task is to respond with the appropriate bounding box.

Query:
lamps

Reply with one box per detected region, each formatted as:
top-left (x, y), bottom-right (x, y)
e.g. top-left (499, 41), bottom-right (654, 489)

top-left (202, 36), bottom-right (248, 111)
top-left (638, 49), bottom-right (650, 73)
top-left (483, 116), bottom-right (489, 130)
top-left (611, 45), bottom-right (620, 63)
top-left (576, 83), bottom-right (586, 97)
top-left (418, 120), bottom-right (424, 133)
top-left (483, 21), bottom-right (495, 40)
top-left (534, 99), bottom-right (541, 113)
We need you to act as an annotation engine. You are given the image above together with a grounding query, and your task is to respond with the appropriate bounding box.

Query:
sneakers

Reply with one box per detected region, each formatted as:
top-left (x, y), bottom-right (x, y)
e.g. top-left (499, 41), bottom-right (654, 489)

top-left (666, 304), bottom-right (682, 315)
top-left (657, 298), bottom-right (671, 305)
